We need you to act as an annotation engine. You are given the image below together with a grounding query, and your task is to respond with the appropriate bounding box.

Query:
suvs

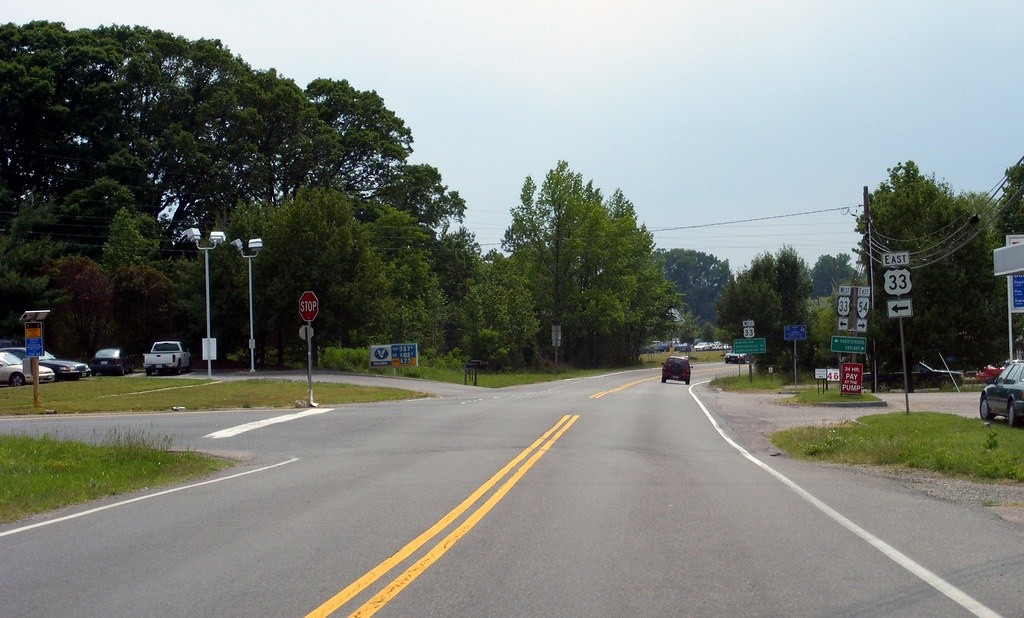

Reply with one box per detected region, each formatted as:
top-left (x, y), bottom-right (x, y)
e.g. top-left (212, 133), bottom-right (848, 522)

top-left (662, 355), bottom-right (693, 384)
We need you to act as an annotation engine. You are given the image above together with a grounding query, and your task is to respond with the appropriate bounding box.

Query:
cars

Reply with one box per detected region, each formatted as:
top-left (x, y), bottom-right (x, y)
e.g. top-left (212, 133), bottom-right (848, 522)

top-left (0, 348), bottom-right (91, 381)
top-left (0, 352), bottom-right (55, 386)
top-left (650, 340), bottom-right (731, 351)
top-left (871, 360), bottom-right (963, 394)
top-left (89, 348), bottom-right (133, 376)
top-left (725, 350), bottom-right (750, 364)
top-left (979, 363), bottom-right (1024, 427)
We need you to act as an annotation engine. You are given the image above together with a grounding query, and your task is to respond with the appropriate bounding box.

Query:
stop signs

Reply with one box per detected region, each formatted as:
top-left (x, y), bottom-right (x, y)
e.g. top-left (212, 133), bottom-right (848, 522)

top-left (299, 291), bottom-right (319, 321)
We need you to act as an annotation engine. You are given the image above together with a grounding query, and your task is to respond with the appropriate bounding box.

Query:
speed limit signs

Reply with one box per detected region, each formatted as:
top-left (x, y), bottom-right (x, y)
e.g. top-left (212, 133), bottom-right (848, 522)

top-left (837, 296), bottom-right (850, 317)
top-left (857, 297), bottom-right (869, 318)
top-left (743, 327), bottom-right (754, 337)
top-left (883, 267), bottom-right (912, 296)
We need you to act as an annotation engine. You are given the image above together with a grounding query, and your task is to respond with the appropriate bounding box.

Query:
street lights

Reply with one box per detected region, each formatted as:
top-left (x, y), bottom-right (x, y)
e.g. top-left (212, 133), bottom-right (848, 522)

top-left (184, 228), bottom-right (226, 378)
top-left (230, 238), bottom-right (263, 372)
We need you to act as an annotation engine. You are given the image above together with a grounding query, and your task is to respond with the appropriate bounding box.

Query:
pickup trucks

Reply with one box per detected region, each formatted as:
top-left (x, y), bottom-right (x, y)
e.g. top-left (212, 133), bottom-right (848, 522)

top-left (142, 341), bottom-right (192, 376)
top-left (977, 366), bottom-right (1005, 384)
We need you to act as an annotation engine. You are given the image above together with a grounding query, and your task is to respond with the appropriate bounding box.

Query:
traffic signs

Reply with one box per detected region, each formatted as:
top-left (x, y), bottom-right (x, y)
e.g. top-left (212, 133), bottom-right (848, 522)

top-left (830, 336), bottom-right (867, 353)
